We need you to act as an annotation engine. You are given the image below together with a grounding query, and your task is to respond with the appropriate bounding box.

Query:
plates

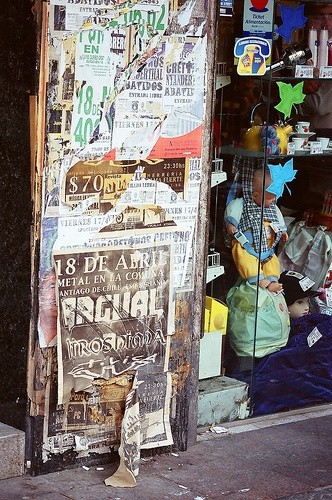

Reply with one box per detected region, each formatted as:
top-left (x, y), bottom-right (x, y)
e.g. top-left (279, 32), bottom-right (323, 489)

top-left (288, 132), bottom-right (315, 138)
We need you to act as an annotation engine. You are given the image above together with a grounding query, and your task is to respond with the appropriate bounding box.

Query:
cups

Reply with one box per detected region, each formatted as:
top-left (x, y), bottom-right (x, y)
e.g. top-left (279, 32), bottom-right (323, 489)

top-left (316, 137), bottom-right (330, 149)
top-left (292, 137), bottom-right (308, 149)
top-left (295, 121), bottom-right (310, 133)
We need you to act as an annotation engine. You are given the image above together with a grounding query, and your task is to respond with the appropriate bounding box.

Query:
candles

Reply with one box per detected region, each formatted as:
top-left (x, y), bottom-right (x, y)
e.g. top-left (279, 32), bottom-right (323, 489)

top-left (309, 26), bottom-right (328, 66)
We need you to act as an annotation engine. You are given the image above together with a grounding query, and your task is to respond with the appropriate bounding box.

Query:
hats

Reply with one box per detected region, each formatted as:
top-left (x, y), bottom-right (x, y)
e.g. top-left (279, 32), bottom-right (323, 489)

top-left (278, 270), bottom-right (317, 305)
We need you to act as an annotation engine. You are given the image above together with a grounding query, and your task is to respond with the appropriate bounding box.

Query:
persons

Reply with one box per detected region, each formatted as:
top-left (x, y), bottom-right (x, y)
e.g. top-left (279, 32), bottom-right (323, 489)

top-left (279, 269), bottom-right (326, 318)
top-left (224, 164), bottom-right (287, 291)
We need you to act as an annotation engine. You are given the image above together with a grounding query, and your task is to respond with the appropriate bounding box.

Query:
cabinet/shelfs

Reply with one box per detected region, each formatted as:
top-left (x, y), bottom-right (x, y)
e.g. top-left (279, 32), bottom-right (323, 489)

top-left (211, 0), bottom-right (332, 416)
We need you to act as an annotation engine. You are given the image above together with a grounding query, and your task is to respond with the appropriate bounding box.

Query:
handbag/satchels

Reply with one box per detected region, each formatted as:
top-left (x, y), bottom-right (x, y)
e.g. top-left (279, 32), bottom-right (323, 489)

top-left (225, 276), bottom-right (291, 358)
